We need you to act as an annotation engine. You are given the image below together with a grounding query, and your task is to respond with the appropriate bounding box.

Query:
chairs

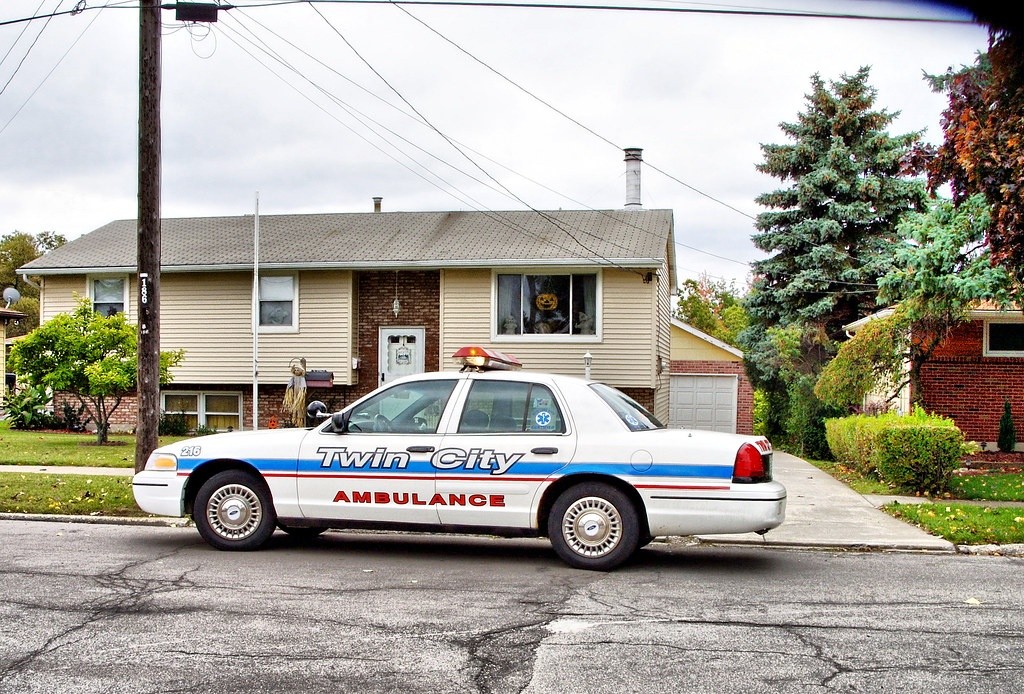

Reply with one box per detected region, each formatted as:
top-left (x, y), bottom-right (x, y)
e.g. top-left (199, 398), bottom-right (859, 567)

top-left (461, 410), bottom-right (489, 433)
top-left (494, 415), bottom-right (516, 432)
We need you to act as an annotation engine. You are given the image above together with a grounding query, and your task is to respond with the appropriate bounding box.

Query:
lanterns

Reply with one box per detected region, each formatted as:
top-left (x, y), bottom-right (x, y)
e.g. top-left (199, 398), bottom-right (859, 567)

top-left (537, 294), bottom-right (558, 309)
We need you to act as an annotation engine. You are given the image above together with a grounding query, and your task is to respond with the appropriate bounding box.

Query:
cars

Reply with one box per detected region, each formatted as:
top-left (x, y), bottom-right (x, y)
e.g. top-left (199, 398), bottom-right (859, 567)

top-left (133, 346), bottom-right (787, 569)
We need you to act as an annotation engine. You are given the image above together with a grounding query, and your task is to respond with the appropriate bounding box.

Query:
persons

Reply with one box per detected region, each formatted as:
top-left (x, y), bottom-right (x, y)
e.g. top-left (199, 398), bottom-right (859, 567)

top-left (504, 316), bottom-right (517, 335)
top-left (282, 365), bottom-right (305, 426)
